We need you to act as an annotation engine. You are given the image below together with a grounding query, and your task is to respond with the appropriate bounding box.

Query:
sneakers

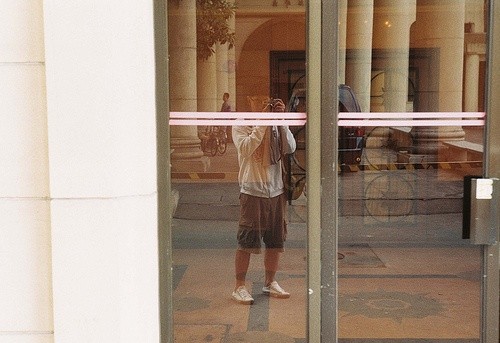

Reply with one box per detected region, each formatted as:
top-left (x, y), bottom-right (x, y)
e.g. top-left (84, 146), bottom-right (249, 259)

top-left (262, 281), bottom-right (290, 299)
top-left (231, 286), bottom-right (254, 305)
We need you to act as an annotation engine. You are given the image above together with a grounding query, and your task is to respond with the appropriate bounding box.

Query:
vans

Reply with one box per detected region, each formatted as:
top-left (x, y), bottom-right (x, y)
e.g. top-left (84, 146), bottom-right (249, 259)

top-left (287, 84), bottom-right (365, 167)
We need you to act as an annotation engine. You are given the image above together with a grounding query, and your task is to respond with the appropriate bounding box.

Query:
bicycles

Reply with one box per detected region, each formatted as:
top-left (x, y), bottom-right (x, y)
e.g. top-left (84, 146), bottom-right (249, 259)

top-left (199, 123), bottom-right (229, 157)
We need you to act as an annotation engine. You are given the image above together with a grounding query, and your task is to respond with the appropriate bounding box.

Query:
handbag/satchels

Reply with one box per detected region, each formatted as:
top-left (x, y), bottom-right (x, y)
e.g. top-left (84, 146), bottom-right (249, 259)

top-left (283, 171), bottom-right (304, 201)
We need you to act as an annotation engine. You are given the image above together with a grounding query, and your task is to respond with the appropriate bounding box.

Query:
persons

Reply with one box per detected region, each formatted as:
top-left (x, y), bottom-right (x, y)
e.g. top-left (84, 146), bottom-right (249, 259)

top-left (230, 82), bottom-right (296, 304)
top-left (221, 93), bottom-right (234, 143)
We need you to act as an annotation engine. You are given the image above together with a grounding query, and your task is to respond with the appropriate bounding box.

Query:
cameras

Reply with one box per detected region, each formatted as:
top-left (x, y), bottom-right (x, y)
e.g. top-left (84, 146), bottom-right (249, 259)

top-left (267, 100), bottom-right (277, 107)
